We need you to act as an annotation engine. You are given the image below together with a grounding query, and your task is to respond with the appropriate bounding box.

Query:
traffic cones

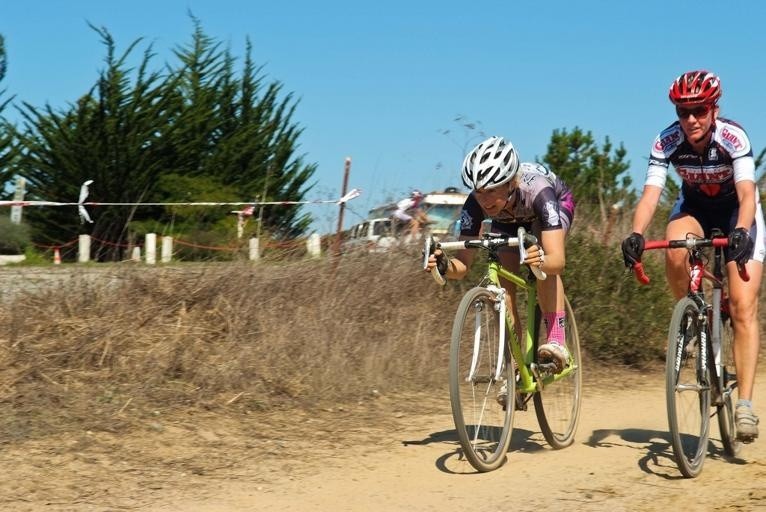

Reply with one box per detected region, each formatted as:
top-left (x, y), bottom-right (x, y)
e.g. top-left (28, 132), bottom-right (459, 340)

top-left (52, 248), bottom-right (62, 264)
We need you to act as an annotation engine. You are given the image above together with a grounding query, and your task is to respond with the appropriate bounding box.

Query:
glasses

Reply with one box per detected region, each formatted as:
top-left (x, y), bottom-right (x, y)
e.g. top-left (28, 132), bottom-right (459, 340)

top-left (676, 104), bottom-right (711, 119)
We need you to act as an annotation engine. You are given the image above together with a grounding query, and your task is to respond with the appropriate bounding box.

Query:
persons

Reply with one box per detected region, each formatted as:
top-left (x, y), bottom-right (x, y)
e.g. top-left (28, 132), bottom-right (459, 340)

top-left (621, 71), bottom-right (765, 439)
top-left (426, 136), bottom-right (575, 407)
top-left (391, 190), bottom-right (430, 244)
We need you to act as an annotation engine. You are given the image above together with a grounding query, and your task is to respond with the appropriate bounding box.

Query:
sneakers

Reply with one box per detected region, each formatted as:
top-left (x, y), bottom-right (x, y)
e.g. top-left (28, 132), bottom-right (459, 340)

top-left (536, 340), bottom-right (568, 374)
top-left (734, 405), bottom-right (761, 439)
top-left (495, 382), bottom-right (528, 413)
top-left (680, 313), bottom-right (708, 356)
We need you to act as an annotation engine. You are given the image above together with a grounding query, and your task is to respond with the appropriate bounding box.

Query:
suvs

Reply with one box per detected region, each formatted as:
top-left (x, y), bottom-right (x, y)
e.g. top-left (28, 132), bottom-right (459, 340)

top-left (339, 218), bottom-right (401, 255)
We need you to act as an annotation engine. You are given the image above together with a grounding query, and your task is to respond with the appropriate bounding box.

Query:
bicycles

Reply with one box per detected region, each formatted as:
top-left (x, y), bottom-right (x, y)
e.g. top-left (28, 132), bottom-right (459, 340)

top-left (420, 224), bottom-right (585, 474)
top-left (629, 233), bottom-right (751, 480)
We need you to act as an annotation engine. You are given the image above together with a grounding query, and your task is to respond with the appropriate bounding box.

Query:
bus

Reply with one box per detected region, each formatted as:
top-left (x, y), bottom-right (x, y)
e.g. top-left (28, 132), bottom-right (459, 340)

top-left (368, 186), bottom-right (468, 244)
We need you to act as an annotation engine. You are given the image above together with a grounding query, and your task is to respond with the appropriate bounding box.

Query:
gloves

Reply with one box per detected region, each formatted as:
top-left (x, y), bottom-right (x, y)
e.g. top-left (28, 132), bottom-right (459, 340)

top-left (729, 228), bottom-right (754, 265)
top-left (621, 232), bottom-right (646, 268)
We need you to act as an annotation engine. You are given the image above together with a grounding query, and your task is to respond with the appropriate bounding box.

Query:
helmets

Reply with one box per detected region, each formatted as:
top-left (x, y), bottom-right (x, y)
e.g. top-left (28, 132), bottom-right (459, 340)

top-left (668, 69), bottom-right (723, 111)
top-left (461, 133), bottom-right (520, 194)
